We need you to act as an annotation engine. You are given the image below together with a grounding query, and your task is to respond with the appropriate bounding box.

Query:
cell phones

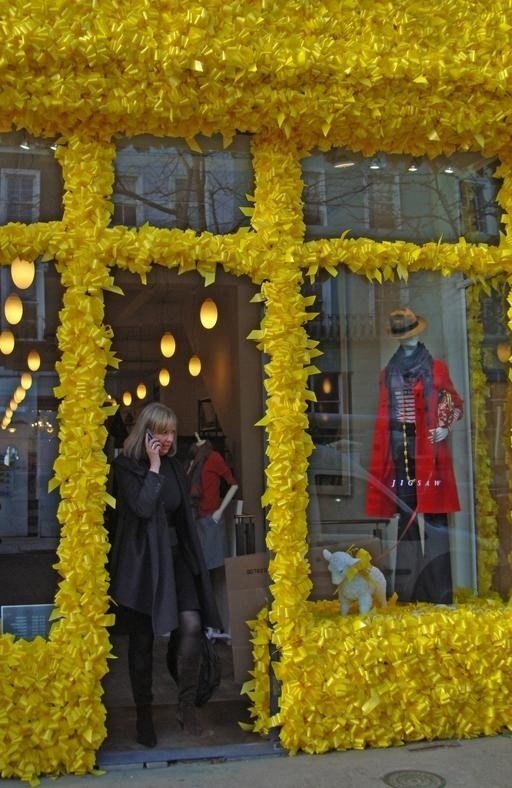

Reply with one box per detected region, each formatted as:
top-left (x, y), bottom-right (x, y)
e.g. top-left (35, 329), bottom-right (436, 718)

top-left (145, 428), bottom-right (153, 441)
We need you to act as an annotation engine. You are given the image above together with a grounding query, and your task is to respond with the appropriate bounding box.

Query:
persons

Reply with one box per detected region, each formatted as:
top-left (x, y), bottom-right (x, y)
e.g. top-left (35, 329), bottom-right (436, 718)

top-left (366, 307), bottom-right (464, 601)
top-left (186, 432), bottom-right (238, 634)
top-left (111, 403), bottom-right (225, 748)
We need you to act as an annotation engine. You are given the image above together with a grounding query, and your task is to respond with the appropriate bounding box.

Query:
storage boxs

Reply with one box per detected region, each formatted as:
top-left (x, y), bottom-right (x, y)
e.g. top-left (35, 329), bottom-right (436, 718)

top-left (225, 537), bottom-right (383, 683)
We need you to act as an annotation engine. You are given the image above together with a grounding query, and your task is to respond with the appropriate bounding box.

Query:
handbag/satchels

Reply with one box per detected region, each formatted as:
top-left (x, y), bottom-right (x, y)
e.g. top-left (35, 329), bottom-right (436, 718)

top-left (166, 627), bottom-right (222, 707)
top-left (438, 390), bottom-right (454, 427)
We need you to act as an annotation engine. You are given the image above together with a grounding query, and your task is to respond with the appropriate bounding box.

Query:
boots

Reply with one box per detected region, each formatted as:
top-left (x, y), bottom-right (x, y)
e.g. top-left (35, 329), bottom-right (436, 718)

top-left (128, 650), bottom-right (157, 747)
top-left (175, 654), bottom-right (203, 738)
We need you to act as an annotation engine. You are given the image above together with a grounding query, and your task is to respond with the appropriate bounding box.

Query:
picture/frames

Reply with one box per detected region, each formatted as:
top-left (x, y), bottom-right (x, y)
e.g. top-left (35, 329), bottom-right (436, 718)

top-left (301, 263), bottom-right (351, 497)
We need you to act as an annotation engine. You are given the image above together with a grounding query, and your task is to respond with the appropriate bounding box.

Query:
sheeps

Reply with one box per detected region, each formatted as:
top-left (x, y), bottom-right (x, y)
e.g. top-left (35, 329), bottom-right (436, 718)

top-left (322, 548), bottom-right (387, 615)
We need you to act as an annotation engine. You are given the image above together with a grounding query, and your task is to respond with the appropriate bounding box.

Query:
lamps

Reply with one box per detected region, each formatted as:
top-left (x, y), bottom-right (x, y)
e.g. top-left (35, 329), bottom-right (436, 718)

top-left (1, 257), bottom-right (40, 431)
top-left (107, 266), bottom-right (219, 408)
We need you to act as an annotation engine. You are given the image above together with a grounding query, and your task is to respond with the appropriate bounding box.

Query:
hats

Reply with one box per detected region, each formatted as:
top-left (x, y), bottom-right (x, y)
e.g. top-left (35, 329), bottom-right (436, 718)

top-left (383, 307), bottom-right (428, 340)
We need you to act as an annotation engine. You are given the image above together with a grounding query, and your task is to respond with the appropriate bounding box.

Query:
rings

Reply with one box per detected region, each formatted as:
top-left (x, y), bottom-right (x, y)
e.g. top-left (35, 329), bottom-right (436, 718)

top-left (152, 444), bottom-right (156, 450)
